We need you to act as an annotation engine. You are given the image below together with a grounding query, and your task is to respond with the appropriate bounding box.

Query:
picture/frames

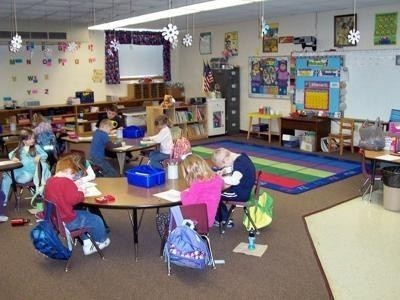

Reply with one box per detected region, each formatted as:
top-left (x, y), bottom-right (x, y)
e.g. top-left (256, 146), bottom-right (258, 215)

top-left (334, 13), bottom-right (357, 47)
top-left (372, 9), bottom-right (400, 50)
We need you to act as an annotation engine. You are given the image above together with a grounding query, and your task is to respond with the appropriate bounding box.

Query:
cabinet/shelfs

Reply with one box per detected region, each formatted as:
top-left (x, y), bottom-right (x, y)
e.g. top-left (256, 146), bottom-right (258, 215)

top-left (209, 66), bottom-right (240, 136)
top-left (127, 81), bottom-right (166, 100)
top-left (146, 104), bottom-right (209, 141)
top-left (0, 95), bottom-right (185, 158)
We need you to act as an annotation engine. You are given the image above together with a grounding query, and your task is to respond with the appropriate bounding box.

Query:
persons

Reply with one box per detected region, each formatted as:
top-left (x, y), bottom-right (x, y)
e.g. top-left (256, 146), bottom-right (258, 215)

top-left (211, 147), bottom-right (256, 229)
top-left (140, 113), bottom-right (174, 170)
top-left (92, 103), bottom-right (133, 166)
top-left (156, 153), bottom-right (223, 255)
top-left (89, 118), bottom-right (120, 178)
top-left (43, 158), bottom-right (111, 257)
top-left (52, 150), bottom-right (111, 244)
top-left (30, 112), bottom-right (59, 166)
top-left (12, 124), bottom-right (54, 216)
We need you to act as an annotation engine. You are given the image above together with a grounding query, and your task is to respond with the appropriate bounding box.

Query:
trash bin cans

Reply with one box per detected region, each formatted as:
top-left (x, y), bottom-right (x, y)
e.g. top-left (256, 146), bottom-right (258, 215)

top-left (381, 166), bottom-right (400, 211)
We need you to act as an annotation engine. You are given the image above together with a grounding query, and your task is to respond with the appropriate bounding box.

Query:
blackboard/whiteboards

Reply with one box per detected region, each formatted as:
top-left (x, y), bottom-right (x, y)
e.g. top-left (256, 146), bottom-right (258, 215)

top-left (249, 55), bottom-right (291, 99)
top-left (291, 48), bottom-right (400, 125)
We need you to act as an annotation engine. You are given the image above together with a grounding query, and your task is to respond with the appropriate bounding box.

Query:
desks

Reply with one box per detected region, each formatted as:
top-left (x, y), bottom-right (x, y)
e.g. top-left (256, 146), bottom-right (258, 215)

top-left (364, 148), bottom-right (400, 204)
top-left (280, 116), bottom-right (331, 152)
top-left (207, 98), bottom-right (226, 136)
top-left (246, 111), bottom-right (283, 144)
top-left (0, 132), bottom-right (190, 262)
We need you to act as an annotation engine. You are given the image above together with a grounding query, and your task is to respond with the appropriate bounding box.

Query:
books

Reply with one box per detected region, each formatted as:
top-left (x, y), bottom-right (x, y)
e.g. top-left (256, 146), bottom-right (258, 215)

top-left (66, 96), bottom-right (81, 106)
top-left (175, 108), bottom-right (207, 139)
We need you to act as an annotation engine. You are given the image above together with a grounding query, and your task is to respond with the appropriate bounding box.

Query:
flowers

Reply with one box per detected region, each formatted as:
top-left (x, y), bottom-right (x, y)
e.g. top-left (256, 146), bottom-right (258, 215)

top-left (224, 51), bottom-right (232, 61)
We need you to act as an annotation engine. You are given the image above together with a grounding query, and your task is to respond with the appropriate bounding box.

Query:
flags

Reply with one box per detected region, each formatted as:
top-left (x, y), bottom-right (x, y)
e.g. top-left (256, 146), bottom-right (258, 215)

top-left (202, 61), bottom-right (211, 95)
top-left (204, 62), bottom-right (215, 84)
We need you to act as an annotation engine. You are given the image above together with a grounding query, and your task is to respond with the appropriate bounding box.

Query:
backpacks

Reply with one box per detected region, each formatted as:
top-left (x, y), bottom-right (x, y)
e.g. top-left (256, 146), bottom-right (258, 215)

top-left (53, 130), bottom-right (68, 161)
top-left (162, 207), bottom-right (210, 271)
top-left (170, 129), bottom-right (192, 160)
top-left (243, 190), bottom-right (274, 232)
top-left (30, 202), bottom-right (73, 259)
top-left (33, 160), bottom-right (51, 195)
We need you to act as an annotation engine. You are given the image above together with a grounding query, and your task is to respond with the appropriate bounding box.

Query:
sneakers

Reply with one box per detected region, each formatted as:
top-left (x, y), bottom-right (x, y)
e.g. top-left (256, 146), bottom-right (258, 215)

top-left (212, 219), bottom-right (233, 227)
top-left (82, 238), bottom-right (111, 255)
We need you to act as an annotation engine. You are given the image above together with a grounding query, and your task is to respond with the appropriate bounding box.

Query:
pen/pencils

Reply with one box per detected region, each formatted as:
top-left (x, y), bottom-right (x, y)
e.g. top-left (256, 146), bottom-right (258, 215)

top-left (167, 160), bottom-right (178, 166)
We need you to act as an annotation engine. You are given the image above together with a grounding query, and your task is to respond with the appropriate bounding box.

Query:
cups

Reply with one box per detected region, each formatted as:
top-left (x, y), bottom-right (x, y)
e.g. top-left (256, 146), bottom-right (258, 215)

top-left (167, 166), bottom-right (179, 180)
top-left (298, 109), bottom-right (342, 119)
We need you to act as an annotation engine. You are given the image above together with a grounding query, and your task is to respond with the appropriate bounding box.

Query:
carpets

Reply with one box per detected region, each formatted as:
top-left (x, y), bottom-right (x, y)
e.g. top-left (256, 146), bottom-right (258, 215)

top-left (191, 139), bottom-right (381, 195)
top-left (302, 186), bottom-right (400, 300)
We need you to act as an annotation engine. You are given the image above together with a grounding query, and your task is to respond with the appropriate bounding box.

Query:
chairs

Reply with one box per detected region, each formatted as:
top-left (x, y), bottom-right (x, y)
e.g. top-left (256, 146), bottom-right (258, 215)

top-left (328, 118), bottom-right (354, 156)
top-left (162, 203), bottom-right (216, 276)
top-left (358, 148), bottom-right (383, 200)
top-left (218, 170), bottom-right (262, 235)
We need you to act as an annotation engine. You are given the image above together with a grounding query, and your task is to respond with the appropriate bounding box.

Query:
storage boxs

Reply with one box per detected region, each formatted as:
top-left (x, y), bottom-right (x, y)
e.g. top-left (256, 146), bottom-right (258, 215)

top-left (282, 134), bottom-right (315, 152)
top-left (76, 92), bottom-right (94, 104)
top-left (209, 57), bottom-right (226, 68)
top-left (251, 123), bottom-right (269, 132)
top-left (88, 106), bottom-right (99, 112)
top-left (64, 97), bottom-right (81, 105)
